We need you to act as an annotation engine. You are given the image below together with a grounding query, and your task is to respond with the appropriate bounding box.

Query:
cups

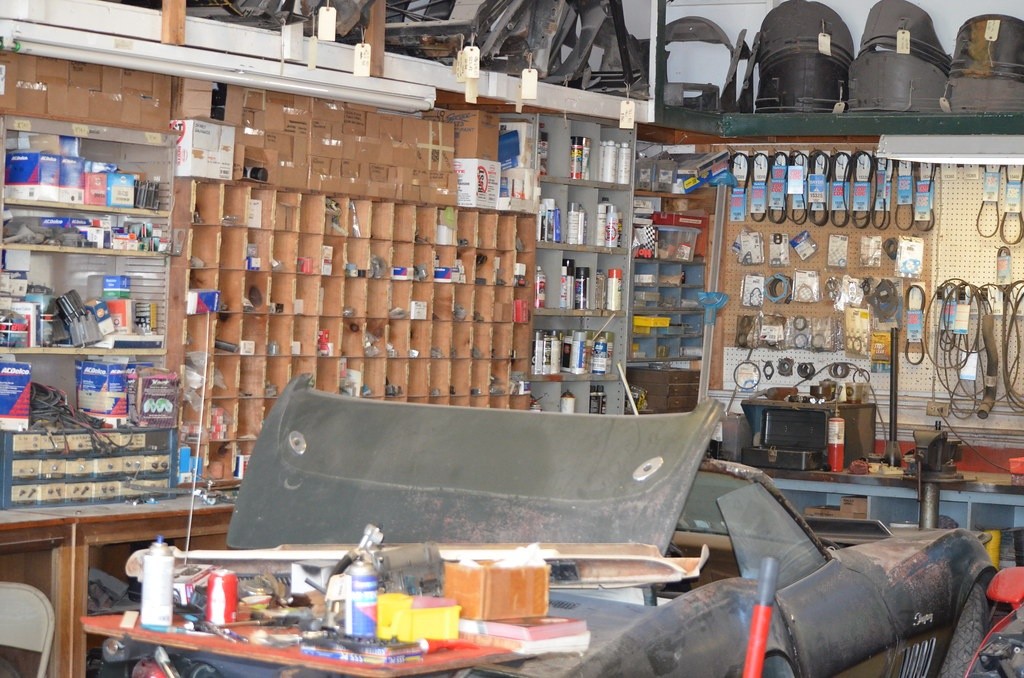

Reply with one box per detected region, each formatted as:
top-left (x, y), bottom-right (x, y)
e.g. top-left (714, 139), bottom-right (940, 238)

top-left (845, 383), bottom-right (863, 404)
top-left (861, 384), bottom-right (870, 403)
top-left (820, 381), bottom-right (836, 400)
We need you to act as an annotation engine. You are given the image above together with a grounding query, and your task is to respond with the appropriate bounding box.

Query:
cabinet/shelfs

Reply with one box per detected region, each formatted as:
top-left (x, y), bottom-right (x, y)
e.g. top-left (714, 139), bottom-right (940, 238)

top-left (0, 114), bottom-right (236, 678)
top-left (632, 138), bottom-right (708, 415)
top-left (500, 112), bottom-right (634, 414)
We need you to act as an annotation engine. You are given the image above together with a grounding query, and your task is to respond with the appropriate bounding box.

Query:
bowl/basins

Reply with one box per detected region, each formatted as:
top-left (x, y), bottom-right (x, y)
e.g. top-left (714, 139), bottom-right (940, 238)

top-left (810, 385), bottom-right (830, 399)
top-left (378, 594), bottom-right (411, 637)
top-left (396, 607), bottom-right (460, 641)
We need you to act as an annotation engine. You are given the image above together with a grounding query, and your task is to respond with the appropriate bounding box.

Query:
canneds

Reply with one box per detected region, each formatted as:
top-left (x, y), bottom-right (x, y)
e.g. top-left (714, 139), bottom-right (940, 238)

top-left (205, 569), bottom-right (238, 624)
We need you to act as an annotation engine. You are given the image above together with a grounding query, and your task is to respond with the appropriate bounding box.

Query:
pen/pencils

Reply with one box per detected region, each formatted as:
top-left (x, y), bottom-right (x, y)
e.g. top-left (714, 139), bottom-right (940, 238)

top-left (142, 624), bottom-right (210, 637)
top-left (206, 621), bottom-right (250, 643)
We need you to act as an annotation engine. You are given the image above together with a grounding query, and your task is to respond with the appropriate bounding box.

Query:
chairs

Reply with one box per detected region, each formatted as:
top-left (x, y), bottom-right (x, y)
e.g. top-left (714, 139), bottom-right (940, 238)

top-left (0, 581), bottom-right (57, 678)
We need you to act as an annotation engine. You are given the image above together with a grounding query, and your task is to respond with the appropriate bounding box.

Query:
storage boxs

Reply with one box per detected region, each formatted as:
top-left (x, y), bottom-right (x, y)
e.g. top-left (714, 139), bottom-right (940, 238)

top-left (75, 354), bottom-right (181, 428)
top-left (441, 556), bottom-right (549, 619)
top-left (234, 453), bottom-right (252, 480)
top-left (409, 300), bottom-right (428, 320)
top-left (0, 353), bottom-right (32, 435)
top-left (635, 196), bottom-right (709, 261)
top-left (0, 248), bottom-right (43, 348)
top-left (423, 111), bottom-right (533, 211)
top-left (391, 266), bottom-right (415, 281)
top-left (663, 152), bottom-right (729, 194)
top-left (0, 51), bottom-right (457, 206)
top-left (187, 289), bottom-right (220, 316)
top-left (83, 276), bottom-right (155, 337)
top-left (804, 496), bottom-right (868, 520)
top-left (434, 267), bottom-right (460, 283)
top-left (5, 131), bottom-right (161, 210)
top-left (3, 216), bottom-right (172, 253)
top-left (634, 157), bottom-right (679, 192)
top-left (174, 175), bottom-right (536, 494)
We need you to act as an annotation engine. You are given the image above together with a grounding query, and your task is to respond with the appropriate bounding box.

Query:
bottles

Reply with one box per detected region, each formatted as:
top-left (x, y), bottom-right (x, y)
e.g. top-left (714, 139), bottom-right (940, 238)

top-left (599, 140), bottom-right (631, 185)
top-left (561, 391), bottom-right (575, 414)
top-left (596, 269), bottom-right (607, 310)
top-left (538, 197), bottom-right (622, 248)
top-left (140, 535), bottom-right (173, 627)
top-left (345, 556), bottom-right (379, 640)
top-left (828, 411), bottom-right (844, 472)
top-left (535, 265), bottom-right (546, 308)
top-left (532, 329), bottom-right (613, 375)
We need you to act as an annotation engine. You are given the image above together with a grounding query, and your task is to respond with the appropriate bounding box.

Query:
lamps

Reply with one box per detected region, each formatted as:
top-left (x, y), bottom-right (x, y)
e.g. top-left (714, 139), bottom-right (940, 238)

top-left (1, 18), bottom-right (439, 113)
top-left (874, 134), bottom-right (1024, 166)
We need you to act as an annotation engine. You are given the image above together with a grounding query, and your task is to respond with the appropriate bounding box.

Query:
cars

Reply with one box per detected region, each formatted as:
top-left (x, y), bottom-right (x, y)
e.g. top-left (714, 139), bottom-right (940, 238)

top-left (98, 372), bottom-right (998, 677)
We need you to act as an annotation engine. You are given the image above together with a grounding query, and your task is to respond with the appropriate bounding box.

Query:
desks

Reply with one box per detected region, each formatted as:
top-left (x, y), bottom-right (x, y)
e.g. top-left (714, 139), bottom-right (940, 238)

top-left (81, 614), bottom-right (538, 676)
